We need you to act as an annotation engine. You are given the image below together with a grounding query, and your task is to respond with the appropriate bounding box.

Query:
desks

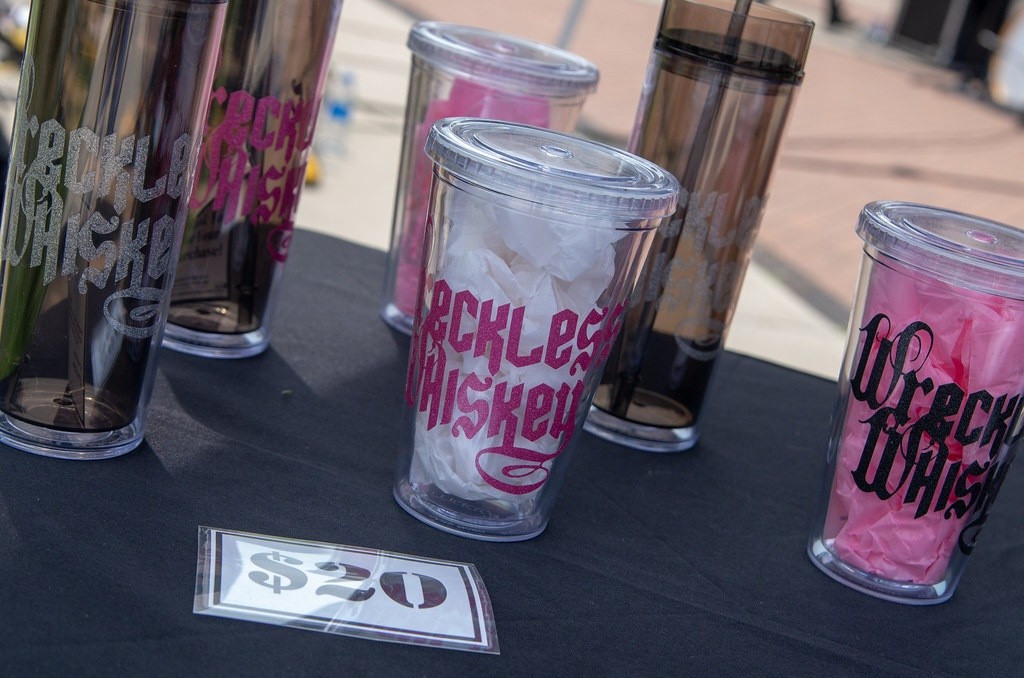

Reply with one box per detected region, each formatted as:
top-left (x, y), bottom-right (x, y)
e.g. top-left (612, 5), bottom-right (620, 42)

top-left (0, 158), bottom-right (1024, 678)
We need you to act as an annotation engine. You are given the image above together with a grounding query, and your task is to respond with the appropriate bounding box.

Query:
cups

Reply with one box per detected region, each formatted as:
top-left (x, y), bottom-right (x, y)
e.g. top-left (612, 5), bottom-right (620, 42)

top-left (379, 20), bottom-right (600, 336)
top-left (391, 118), bottom-right (679, 543)
top-left (0, 0), bottom-right (229, 459)
top-left (161, 0), bottom-right (344, 359)
top-left (810, 202), bottom-right (1024, 604)
top-left (584, 1), bottom-right (815, 452)
